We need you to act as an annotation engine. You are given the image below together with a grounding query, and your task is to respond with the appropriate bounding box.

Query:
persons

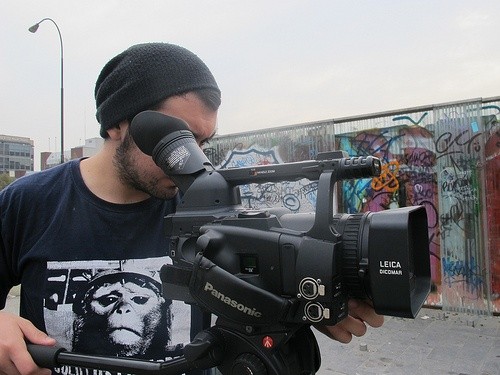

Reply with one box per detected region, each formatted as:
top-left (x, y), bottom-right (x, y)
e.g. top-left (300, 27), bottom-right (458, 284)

top-left (1, 43), bottom-right (384, 375)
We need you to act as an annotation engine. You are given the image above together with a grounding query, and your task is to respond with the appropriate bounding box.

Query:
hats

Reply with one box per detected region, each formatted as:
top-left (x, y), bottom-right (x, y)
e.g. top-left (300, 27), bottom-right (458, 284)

top-left (93, 41), bottom-right (221, 139)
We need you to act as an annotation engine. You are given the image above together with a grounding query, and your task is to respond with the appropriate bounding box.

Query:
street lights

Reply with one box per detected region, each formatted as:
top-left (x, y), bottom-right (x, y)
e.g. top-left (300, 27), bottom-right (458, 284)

top-left (28, 17), bottom-right (66, 164)
